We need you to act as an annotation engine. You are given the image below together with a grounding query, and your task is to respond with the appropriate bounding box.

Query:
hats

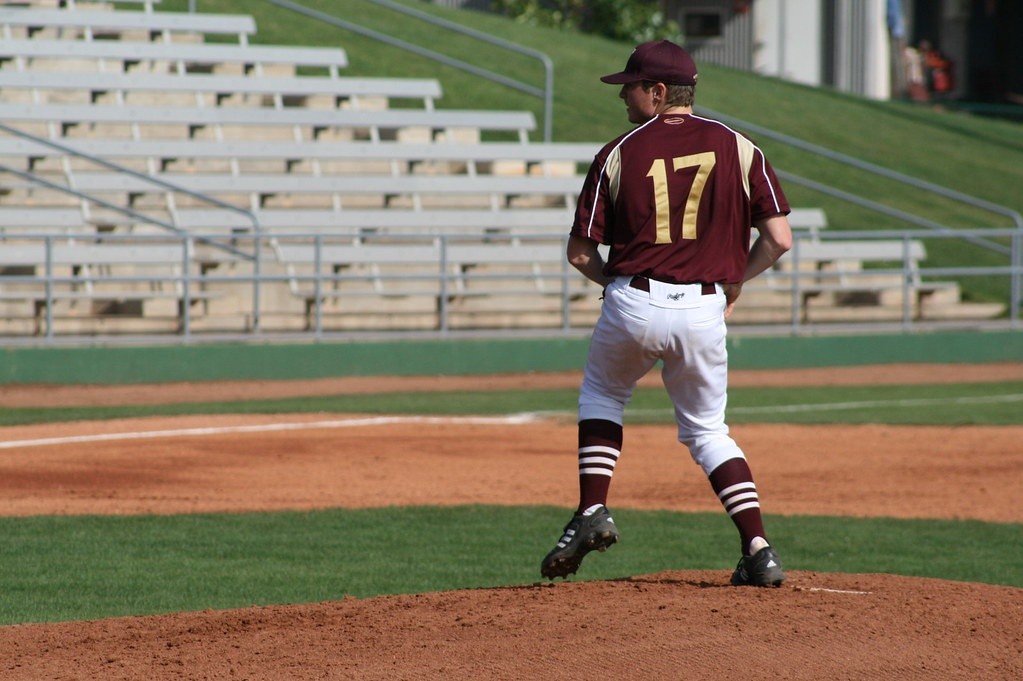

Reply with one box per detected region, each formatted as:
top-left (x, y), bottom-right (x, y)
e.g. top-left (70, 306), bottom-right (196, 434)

top-left (601, 40), bottom-right (697, 85)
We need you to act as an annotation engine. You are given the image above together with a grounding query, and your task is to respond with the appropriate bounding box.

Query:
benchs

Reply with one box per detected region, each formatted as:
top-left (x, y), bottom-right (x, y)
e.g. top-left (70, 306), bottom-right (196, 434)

top-left (0, 0), bottom-right (1010, 336)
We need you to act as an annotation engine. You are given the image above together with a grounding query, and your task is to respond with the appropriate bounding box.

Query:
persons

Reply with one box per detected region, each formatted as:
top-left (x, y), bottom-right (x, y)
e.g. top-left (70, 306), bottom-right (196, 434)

top-left (542, 40), bottom-right (793, 584)
top-left (905, 40), bottom-right (947, 95)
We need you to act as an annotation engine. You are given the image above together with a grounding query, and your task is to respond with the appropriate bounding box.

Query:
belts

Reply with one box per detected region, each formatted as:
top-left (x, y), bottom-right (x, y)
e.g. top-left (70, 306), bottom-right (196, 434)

top-left (630, 276), bottom-right (717, 295)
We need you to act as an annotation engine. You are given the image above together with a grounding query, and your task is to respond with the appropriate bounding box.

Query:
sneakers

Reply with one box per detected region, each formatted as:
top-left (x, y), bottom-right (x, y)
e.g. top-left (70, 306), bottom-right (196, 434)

top-left (540, 507), bottom-right (619, 581)
top-left (729, 546), bottom-right (784, 586)
